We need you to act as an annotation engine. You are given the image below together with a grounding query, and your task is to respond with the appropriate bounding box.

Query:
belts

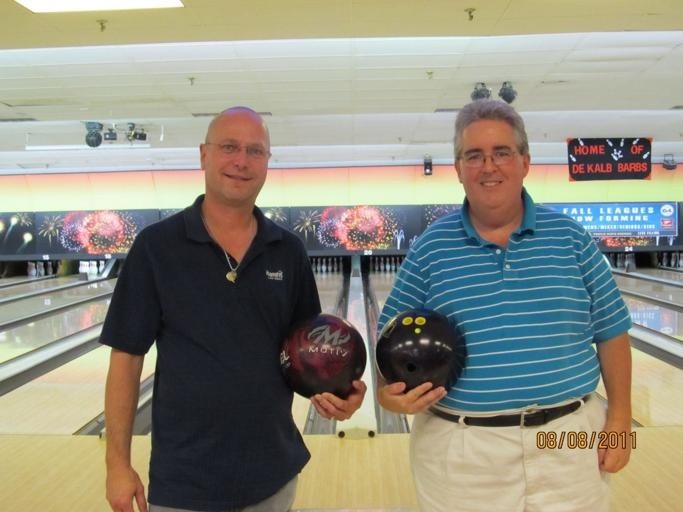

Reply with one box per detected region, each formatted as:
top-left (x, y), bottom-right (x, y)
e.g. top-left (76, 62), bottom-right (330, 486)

top-left (428, 394), bottom-right (593, 427)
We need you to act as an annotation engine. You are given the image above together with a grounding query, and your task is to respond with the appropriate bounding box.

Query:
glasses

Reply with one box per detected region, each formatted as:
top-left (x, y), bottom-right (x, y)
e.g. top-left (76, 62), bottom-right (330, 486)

top-left (207, 142), bottom-right (272, 160)
top-left (461, 149), bottom-right (520, 168)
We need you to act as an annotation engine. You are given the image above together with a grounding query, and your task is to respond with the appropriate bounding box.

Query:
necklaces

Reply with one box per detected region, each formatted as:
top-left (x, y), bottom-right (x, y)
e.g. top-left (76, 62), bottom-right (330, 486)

top-left (198, 204), bottom-right (260, 284)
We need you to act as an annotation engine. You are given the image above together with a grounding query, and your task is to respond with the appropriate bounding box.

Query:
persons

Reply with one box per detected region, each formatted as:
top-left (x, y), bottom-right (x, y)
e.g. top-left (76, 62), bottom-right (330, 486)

top-left (373, 95), bottom-right (635, 510)
top-left (101, 103), bottom-right (369, 511)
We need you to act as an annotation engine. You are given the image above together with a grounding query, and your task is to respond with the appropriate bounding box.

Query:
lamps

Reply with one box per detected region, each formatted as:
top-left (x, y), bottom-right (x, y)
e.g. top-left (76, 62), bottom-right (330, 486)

top-left (421, 154), bottom-right (433, 177)
top-left (83, 122), bottom-right (148, 149)
top-left (662, 153), bottom-right (677, 171)
top-left (467, 81), bottom-right (520, 104)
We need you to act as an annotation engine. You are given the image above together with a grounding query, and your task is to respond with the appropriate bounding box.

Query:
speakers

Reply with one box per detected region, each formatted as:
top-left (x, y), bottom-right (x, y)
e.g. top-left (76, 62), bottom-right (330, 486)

top-left (424, 159), bottom-right (432, 175)
